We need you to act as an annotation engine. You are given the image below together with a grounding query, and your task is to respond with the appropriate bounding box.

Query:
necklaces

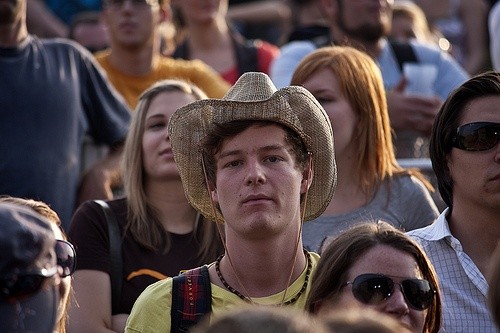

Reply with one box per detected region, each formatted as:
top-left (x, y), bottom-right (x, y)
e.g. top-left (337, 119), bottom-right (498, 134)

top-left (215, 248), bottom-right (312, 308)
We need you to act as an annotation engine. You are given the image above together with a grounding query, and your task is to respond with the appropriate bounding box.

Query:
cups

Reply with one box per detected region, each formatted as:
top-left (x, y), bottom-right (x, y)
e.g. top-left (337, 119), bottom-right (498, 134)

top-left (404, 64), bottom-right (437, 99)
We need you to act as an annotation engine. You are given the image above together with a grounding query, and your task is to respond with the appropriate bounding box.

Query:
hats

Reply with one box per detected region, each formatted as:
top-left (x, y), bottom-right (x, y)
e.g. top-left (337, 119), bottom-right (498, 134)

top-left (166, 72), bottom-right (338, 224)
top-left (0, 206), bottom-right (60, 333)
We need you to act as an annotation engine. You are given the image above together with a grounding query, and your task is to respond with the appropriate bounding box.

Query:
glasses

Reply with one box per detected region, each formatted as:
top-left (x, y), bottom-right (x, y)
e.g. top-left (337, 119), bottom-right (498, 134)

top-left (444, 122), bottom-right (500, 153)
top-left (52, 240), bottom-right (77, 280)
top-left (334, 273), bottom-right (438, 311)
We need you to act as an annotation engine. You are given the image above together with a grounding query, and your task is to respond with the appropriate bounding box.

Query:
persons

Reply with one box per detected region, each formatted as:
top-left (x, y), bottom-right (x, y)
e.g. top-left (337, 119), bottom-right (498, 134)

top-left (0, 0), bottom-right (500, 333)
